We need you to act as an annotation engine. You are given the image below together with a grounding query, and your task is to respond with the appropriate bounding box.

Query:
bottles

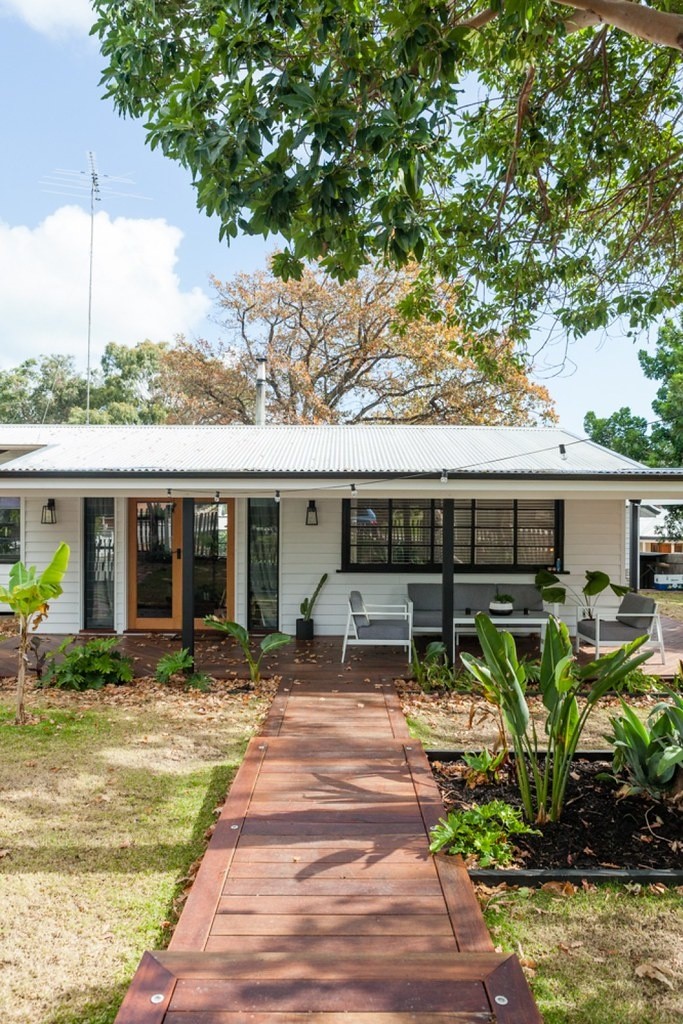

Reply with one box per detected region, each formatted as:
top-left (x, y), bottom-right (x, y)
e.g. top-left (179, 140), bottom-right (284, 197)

top-left (556, 558), bottom-right (561, 571)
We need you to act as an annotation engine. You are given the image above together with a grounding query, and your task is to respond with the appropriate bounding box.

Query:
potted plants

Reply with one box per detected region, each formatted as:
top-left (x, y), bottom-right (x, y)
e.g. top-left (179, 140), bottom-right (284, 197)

top-left (536, 566), bottom-right (634, 644)
top-left (296, 573), bottom-right (328, 640)
top-left (489, 593), bottom-right (516, 616)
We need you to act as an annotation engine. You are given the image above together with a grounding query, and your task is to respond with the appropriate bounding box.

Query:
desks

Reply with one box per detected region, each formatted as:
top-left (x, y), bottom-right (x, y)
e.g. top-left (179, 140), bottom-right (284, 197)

top-left (452, 612), bottom-right (559, 665)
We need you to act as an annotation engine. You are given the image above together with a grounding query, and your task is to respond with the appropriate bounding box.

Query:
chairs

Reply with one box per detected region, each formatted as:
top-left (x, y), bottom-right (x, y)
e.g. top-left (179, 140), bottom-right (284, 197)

top-left (341, 591), bottom-right (413, 664)
top-left (576, 593), bottom-right (665, 665)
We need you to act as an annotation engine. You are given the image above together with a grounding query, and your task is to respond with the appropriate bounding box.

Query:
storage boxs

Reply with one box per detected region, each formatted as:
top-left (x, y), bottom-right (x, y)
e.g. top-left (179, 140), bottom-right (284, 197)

top-left (654, 574), bottom-right (683, 591)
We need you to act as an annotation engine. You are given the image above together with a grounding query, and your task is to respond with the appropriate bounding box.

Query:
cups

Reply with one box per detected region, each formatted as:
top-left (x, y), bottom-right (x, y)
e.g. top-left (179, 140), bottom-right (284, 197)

top-left (524, 608), bottom-right (529, 615)
top-left (465, 608), bottom-right (471, 615)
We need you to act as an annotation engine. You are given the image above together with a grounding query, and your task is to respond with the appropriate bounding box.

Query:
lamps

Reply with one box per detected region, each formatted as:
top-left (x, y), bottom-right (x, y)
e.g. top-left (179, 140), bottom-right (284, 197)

top-left (305, 500), bottom-right (319, 526)
top-left (41, 499), bottom-right (57, 524)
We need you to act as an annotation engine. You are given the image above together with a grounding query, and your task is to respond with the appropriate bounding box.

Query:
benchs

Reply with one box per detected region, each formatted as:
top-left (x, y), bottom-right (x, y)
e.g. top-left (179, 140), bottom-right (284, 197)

top-left (405, 582), bottom-right (559, 649)
